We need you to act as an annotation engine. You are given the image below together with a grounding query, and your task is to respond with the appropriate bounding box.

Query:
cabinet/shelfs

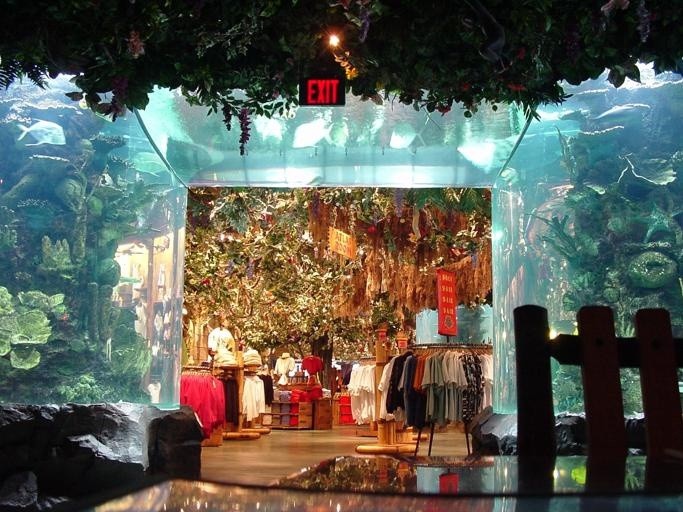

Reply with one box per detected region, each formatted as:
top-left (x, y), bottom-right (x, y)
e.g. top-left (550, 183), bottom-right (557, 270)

top-left (313, 398), bottom-right (334, 430)
top-left (262, 401), bottom-right (312, 430)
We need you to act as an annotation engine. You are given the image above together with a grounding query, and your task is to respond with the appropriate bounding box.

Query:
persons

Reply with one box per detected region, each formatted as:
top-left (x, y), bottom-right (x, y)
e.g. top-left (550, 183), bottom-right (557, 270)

top-left (207, 322), bottom-right (235, 364)
